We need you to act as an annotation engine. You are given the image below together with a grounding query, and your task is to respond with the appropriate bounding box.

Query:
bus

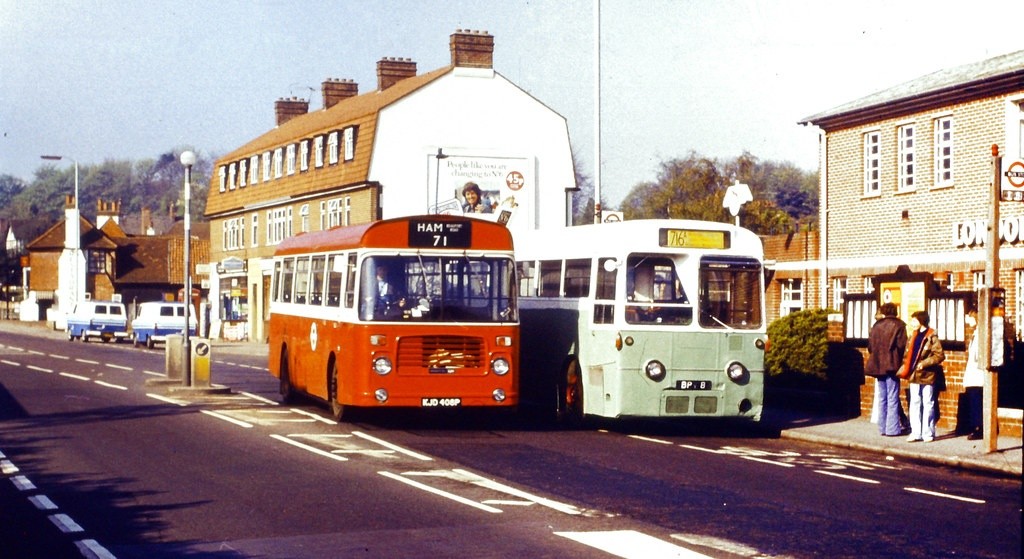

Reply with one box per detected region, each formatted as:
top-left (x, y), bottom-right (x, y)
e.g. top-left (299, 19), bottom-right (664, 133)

top-left (442, 217), bottom-right (768, 426)
top-left (266, 214), bottom-right (519, 422)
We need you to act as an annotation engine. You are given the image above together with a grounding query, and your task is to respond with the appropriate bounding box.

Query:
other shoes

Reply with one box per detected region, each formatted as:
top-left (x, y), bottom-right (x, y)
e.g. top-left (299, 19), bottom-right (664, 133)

top-left (906, 437), bottom-right (922, 442)
top-left (968, 426), bottom-right (982, 439)
top-left (924, 437), bottom-right (933, 442)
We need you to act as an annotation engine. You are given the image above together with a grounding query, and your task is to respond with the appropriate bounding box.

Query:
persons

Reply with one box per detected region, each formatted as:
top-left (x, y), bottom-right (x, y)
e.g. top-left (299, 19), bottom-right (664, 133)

top-left (864, 302), bottom-right (913, 438)
top-left (364, 262), bottom-right (406, 311)
top-left (961, 294), bottom-right (985, 440)
top-left (461, 182), bottom-right (492, 213)
top-left (895, 309), bottom-right (946, 443)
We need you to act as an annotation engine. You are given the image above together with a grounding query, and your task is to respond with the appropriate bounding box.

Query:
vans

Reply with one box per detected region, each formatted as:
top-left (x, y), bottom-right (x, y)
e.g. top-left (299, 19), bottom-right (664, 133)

top-left (130, 302), bottom-right (198, 349)
top-left (65, 301), bottom-right (127, 344)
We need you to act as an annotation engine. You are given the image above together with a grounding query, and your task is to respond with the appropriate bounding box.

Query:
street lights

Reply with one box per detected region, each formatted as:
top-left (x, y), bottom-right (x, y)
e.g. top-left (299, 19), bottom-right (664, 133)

top-left (180, 149), bottom-right (195, 385)
top-left (40, 154), bottom-right (80, 302)
